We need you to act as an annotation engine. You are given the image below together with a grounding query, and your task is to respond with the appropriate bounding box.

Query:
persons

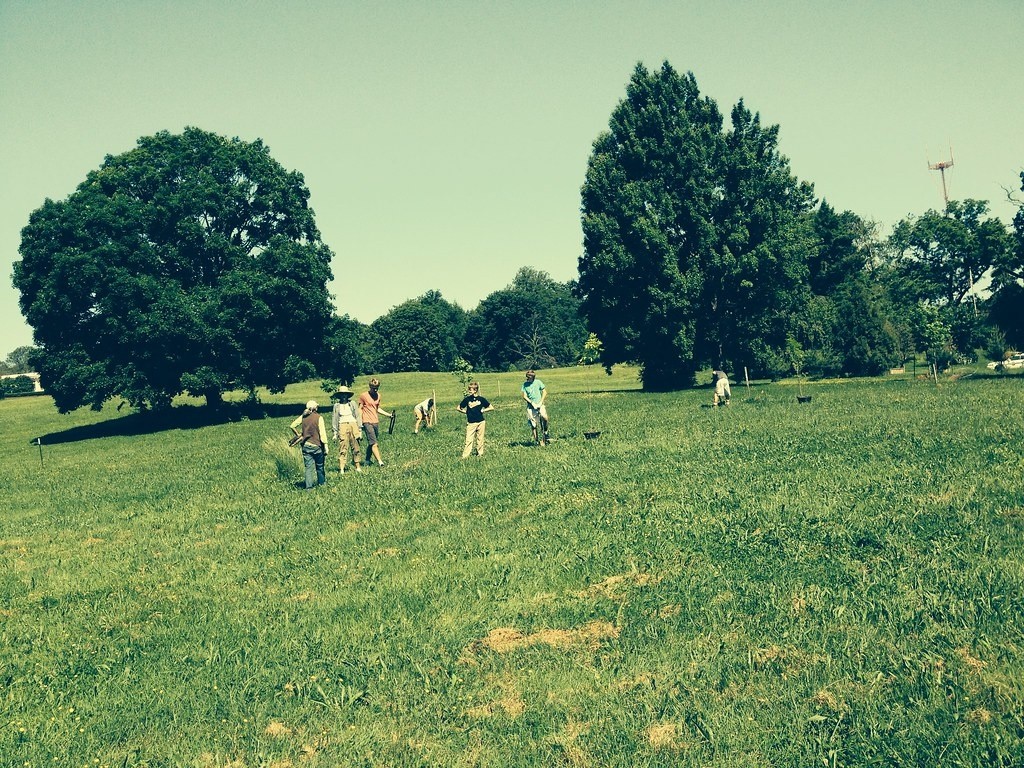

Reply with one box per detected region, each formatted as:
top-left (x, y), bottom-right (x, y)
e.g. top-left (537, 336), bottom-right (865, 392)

top-left (332, 386), bottom-right (363, 474)
top-left (456, 382), bottom-right (494, 459)
top-left (522, 370), bottom-right (550, 445)
top-left (712, 364), bottom-right (731, 405)
top-left (289, 400), bottom-right (330, 489)
top-left (360, 379), bottom-right (393, 466)
top-left (414, 389), bottom-right (435, 433)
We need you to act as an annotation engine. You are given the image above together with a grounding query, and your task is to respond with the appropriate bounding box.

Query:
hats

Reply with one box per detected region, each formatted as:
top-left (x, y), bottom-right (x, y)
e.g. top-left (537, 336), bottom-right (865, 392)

top-left (334, 385), bottom-right (354, 398)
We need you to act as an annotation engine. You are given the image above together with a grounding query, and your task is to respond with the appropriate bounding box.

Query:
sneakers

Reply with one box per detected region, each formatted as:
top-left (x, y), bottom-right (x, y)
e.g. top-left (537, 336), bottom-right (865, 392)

top-left (379, 461), bottom-right (384, 466)
